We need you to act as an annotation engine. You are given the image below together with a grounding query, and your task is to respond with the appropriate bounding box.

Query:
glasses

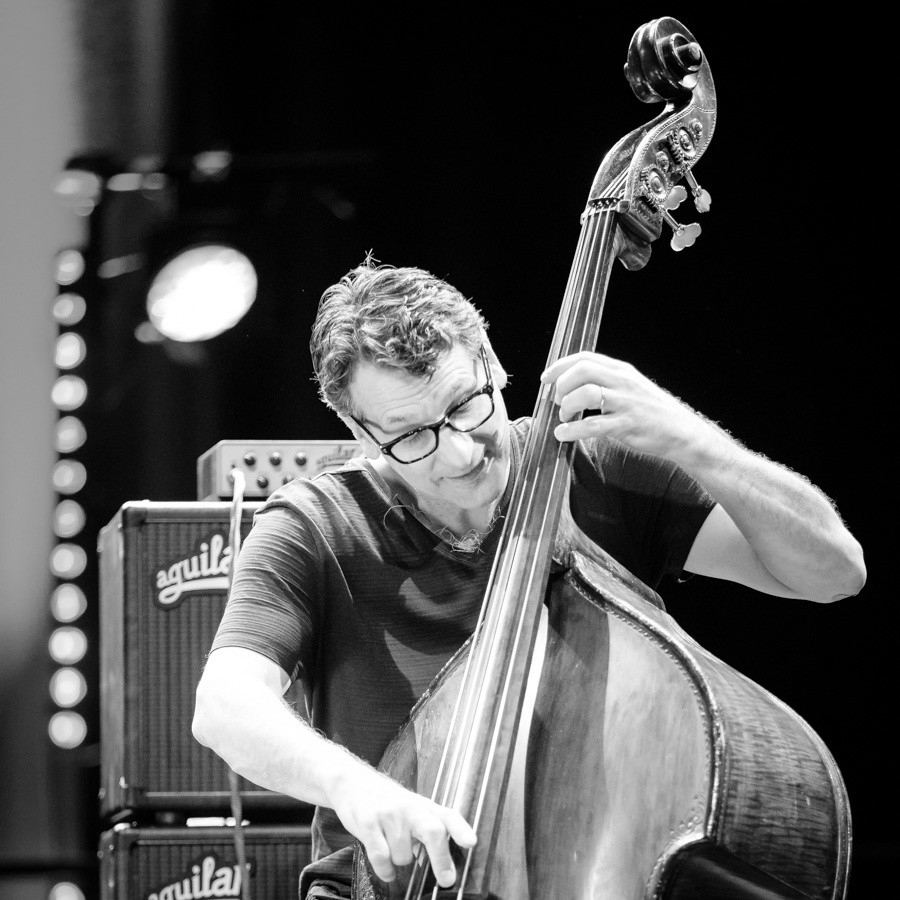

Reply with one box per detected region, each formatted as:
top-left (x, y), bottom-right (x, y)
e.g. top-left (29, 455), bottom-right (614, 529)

top-left (349, 343), bottom-right (495, 465)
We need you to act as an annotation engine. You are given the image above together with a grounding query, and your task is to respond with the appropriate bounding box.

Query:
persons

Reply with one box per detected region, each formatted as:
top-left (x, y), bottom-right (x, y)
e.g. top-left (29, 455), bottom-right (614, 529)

top-left (192, 249), bottom-right (867, 900)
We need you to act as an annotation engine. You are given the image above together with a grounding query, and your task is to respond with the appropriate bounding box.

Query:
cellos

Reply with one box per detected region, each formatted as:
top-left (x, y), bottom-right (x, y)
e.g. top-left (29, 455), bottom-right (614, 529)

top-left (348, 12), bottom-right (857, 900)
